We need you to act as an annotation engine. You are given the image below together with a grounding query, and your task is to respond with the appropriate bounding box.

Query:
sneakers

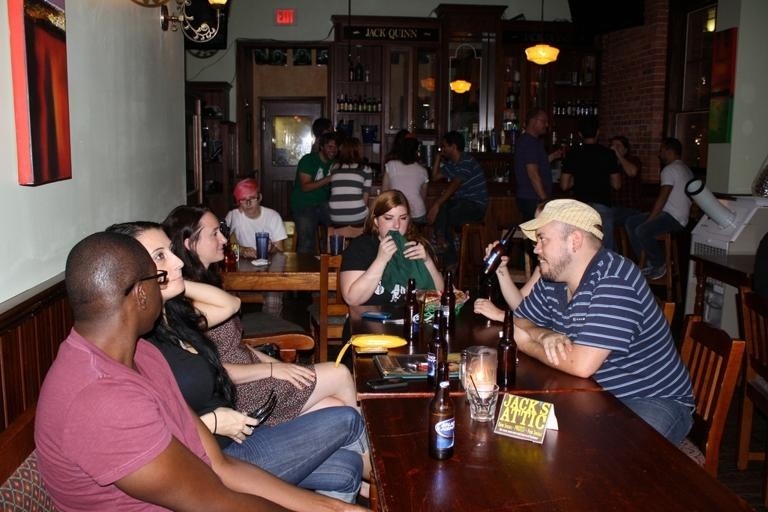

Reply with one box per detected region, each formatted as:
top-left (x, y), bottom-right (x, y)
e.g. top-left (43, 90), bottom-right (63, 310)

top-left (652, 259), bottom-right (674, 280)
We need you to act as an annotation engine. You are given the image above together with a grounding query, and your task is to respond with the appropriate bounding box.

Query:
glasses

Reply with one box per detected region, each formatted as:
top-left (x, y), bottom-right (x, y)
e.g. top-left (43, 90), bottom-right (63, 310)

top-left (237, 192), bottom-right (260, 204)
top-left (122, 269), bottom-right (168, 296)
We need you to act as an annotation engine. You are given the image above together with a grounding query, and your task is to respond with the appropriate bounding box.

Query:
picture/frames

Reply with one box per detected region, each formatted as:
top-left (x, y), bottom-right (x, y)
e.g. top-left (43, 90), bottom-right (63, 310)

top-left (9, 0), bottom-right (72, 187)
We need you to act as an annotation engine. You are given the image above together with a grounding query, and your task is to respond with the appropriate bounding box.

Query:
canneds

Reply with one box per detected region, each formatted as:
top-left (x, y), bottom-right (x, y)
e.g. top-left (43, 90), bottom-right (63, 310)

top-left (560, 144), bottom-right (569, 159)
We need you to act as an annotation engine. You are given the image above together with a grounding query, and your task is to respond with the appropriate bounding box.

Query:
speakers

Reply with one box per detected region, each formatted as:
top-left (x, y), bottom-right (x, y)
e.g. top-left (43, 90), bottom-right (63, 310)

top-left (568, 0), bottom-right (644, 33)
top-left (184, 0), bottom-right (227, 50)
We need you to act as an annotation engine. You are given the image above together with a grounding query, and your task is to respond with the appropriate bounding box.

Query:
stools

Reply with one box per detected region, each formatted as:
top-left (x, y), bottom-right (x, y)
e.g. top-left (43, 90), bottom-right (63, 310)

top-left (501, 229), bottom-right (530, 285)
top-left (638, 233), bottom-right (683, 305)
top-left (456, 223), bottom-right (485, 290)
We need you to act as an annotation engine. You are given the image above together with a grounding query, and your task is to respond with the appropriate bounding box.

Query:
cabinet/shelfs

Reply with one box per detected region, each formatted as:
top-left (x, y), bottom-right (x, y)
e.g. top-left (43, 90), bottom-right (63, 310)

top-left (328, 3), bottom-right (601, 181)
top-left (674, 4), bottom-right (719, 182)
top-left (185, 80), bottom-right (233, 220)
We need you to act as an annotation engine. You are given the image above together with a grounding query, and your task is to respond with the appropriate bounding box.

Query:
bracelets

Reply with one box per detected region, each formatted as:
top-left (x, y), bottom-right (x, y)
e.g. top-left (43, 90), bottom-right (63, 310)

top-left (269, 362), bottom-right (274, 379)
top-left (209, 411), bottom-right (217, 437)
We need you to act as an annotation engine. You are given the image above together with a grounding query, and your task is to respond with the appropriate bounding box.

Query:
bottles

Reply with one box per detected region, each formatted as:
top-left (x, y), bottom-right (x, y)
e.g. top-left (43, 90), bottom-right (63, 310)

top-left (349, 55), bottom-right (370, 82)
top-left (337, 90), bottom-right (382, 113)
top-left (429, 361), bottom-right (455, 459)
top-left (427, 310), bottom-right (450, 388)
top-left (552, 127), bottom-right (558, 145)
top-left (404, 279), bottom-right (421, 340)
top-left (466, 119), bottom-right (518, 153)
top-left (422, 111), bottom-right (431, 130)
top-left (552, 98), bottom-right (597, 116)
top-left (459, 351), bottom-right (465, 381)
top-left (502, 62), bottom-right (521, 109)
top-left (497, 310), bottom-right (518, 388)
top-left (478, 226), bottom-right (516, 278)
top-left (229, 231), bottom-right (240, 261)
top-left (441, 270), bottom-right (456, 330)
top-left (222, 227), bottom-right (236, 266)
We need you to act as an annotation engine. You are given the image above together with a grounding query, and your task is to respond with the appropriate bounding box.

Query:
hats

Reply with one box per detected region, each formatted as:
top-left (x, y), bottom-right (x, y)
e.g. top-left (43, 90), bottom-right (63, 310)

top-left (517, 196), bottom-right (606, 244)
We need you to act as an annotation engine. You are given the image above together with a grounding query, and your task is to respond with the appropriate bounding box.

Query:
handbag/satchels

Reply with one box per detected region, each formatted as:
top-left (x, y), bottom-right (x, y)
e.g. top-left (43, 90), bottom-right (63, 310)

top-left (253, 343), bottom-right (282, 360)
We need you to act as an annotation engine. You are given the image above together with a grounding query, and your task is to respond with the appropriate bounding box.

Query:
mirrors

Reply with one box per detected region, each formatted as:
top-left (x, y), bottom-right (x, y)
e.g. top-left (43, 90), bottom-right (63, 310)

top-left (448, 31), bottom-right (495, 150)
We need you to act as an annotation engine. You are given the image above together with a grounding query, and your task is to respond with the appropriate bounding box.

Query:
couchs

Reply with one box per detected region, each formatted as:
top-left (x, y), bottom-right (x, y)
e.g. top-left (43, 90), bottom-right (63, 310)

top-left (0, 269), bottom-right (315, 512)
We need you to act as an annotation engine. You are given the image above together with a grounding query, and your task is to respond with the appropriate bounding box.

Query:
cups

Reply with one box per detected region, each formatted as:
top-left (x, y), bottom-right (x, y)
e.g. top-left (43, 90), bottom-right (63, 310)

top-left (467, 382), bottom-right (498, 421)
top-left (560, 138), bottom-right (569, 159)
top-left (330, 235), bottom-right (344, 256)
top-left (255, 231), bottom-right (269, 259)
top-left (568, 127), bottom-right (576, 140)
top-left (568, 72), bottom-right (579, 86)
top-left (465, 346), bottom-right (498, 399)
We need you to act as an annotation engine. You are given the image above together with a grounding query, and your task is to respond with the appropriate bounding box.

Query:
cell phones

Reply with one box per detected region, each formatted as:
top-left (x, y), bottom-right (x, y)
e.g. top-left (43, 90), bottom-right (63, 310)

top-left (361, 311), bottom-right (392, 320)
top-left (365, 377), bottom-right (410, 390)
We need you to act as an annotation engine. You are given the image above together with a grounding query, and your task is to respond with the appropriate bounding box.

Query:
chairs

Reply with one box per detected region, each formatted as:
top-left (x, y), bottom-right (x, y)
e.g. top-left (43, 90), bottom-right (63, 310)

top-left (654, 294), bottom-right (676, 326)
top-left (737, 285), bottom-right (768, 471)
top-left (678, 314), bottom-right (746, 477)
top-left (227, 221), bottom-right (297, 304)
top-left (327, 226), bottom-right (365, 256)
top-left (306, 252), bottom-right (350, 364)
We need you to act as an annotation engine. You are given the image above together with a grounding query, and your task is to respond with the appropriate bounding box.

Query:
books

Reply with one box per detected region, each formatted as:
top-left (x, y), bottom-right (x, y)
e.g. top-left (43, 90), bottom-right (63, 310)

top-left (373, 350), bottom-right (462, 380)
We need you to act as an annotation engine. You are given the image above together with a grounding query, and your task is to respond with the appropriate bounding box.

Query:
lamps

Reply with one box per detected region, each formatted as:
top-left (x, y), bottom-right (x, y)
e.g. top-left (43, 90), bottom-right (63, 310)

top-left (524, 0), bottom-right (561, 66)
top-left (449, 80), bottom-right (472, 95)
top-left (129, 0), bottom-right (228, 44)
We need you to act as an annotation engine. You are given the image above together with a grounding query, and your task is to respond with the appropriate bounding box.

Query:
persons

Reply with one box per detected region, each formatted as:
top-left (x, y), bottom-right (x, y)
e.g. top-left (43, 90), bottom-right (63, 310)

top-left (106, 220), bottom-right (373, 504)
top-left (338, 187), bottom-right (444, 305)
top-left (30, 231), bottom-right (385, 512)
top-left (221, 179), bottom-right (287, 317)
top-left (471, 198), bottom-right (558, 323)
top-left (289, 107), bottom-right (696, 283)
top-left (160, 202), bottom-right (363, 428)
top-left (512, 198), bottom-right (696, 445)
